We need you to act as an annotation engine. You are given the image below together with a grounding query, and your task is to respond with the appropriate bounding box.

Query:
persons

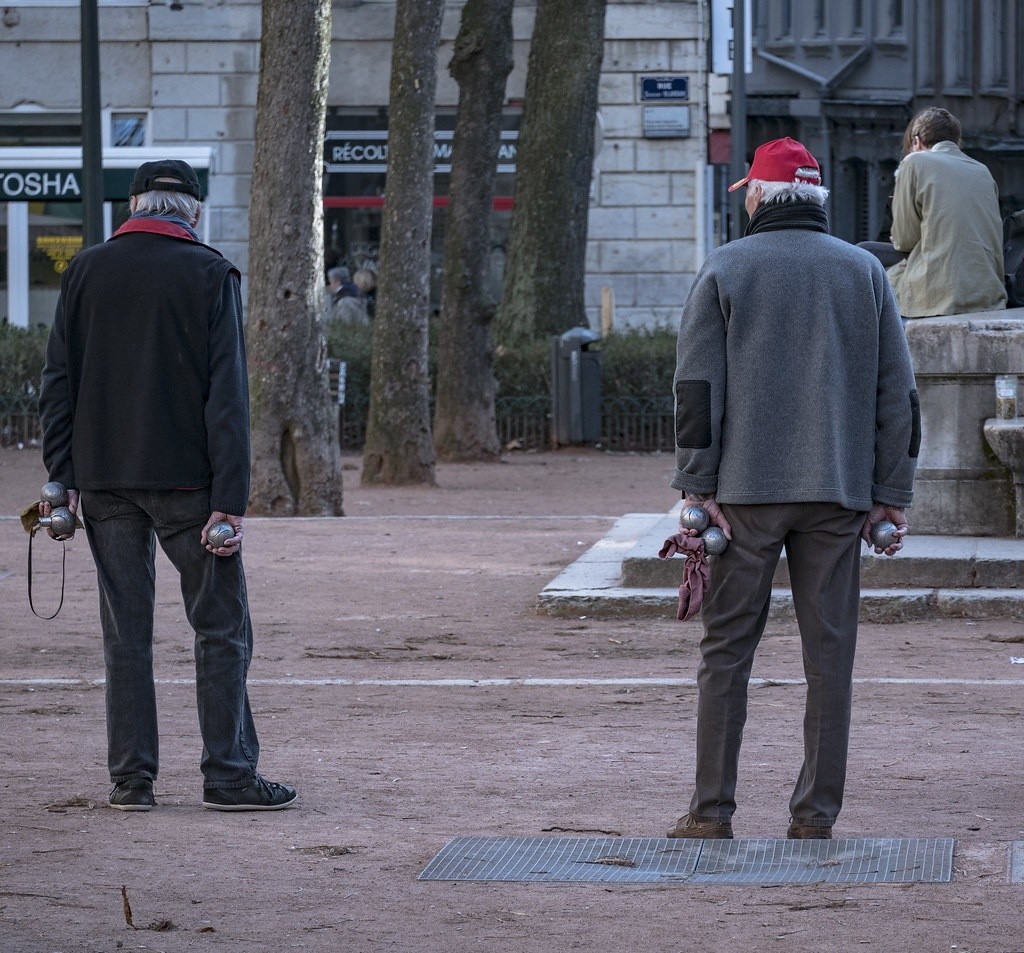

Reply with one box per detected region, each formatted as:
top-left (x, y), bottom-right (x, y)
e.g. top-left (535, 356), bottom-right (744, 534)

top-left (852, 105), bottom-right (1008, 319)
top-left (665, 136), bottom-right (924, 840)
top-left (327, 266), bottom-right (378, 315)
top-left (36, 156), bottom-right (302, 812)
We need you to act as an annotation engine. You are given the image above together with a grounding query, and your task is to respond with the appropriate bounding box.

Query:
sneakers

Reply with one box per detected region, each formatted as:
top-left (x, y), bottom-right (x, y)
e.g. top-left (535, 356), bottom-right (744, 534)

top-left (201, 776), bottom-right (296, 812)
top-left (666, 814), bottom-right (735, 840)
top-left (109, 775), bottom-right (154, 810)
top-left (785, 821), bottom-right (833, 840)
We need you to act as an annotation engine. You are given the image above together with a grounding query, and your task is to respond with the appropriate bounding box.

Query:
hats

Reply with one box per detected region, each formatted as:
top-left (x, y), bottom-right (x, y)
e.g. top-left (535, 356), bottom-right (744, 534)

top-left (728, 137), bottom-right (821, 193)
top-left (129, 159), bottom-right (201, 200)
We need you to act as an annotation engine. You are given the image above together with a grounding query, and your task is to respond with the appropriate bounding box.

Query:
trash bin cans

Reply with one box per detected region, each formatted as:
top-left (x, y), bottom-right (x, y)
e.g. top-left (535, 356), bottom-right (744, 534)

top-left (551, 327), bottom-right (602, 451)
